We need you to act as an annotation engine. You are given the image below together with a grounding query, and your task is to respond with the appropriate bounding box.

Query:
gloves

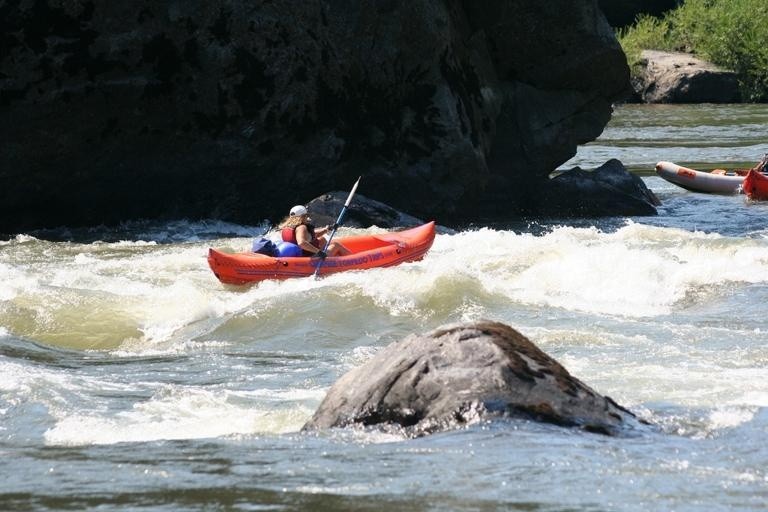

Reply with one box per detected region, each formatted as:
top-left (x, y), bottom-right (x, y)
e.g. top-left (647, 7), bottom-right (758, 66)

top-left (327, 223), bottom-right (338, 231)
top-left (317, 250), bottom-right (326, 258)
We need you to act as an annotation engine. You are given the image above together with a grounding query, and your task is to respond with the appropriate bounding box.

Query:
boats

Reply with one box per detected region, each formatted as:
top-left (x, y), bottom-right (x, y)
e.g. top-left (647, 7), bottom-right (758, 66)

top-left (208, 221), bottom-right (435, 284)
top-left (656, 161), bottom-right (768, 199)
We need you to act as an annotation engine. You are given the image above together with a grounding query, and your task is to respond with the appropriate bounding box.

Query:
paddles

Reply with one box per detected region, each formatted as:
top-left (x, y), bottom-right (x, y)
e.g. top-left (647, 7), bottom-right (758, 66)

top-left (314, 176), bottom-right (361, 278)
top-left (712, 168), bottom-right (738, 175)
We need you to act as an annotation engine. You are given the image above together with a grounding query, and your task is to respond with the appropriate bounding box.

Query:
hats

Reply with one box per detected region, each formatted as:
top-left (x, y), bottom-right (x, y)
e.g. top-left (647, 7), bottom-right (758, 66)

top-left (290, 204), bottom-right (307, 217)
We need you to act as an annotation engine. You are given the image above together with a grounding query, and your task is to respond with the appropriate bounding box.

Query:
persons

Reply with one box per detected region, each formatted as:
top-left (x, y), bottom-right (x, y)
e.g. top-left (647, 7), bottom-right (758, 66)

top-left (753, 153), bottom-right (768, 172)
top-left (281, 205), bottom-right (354, 258)
